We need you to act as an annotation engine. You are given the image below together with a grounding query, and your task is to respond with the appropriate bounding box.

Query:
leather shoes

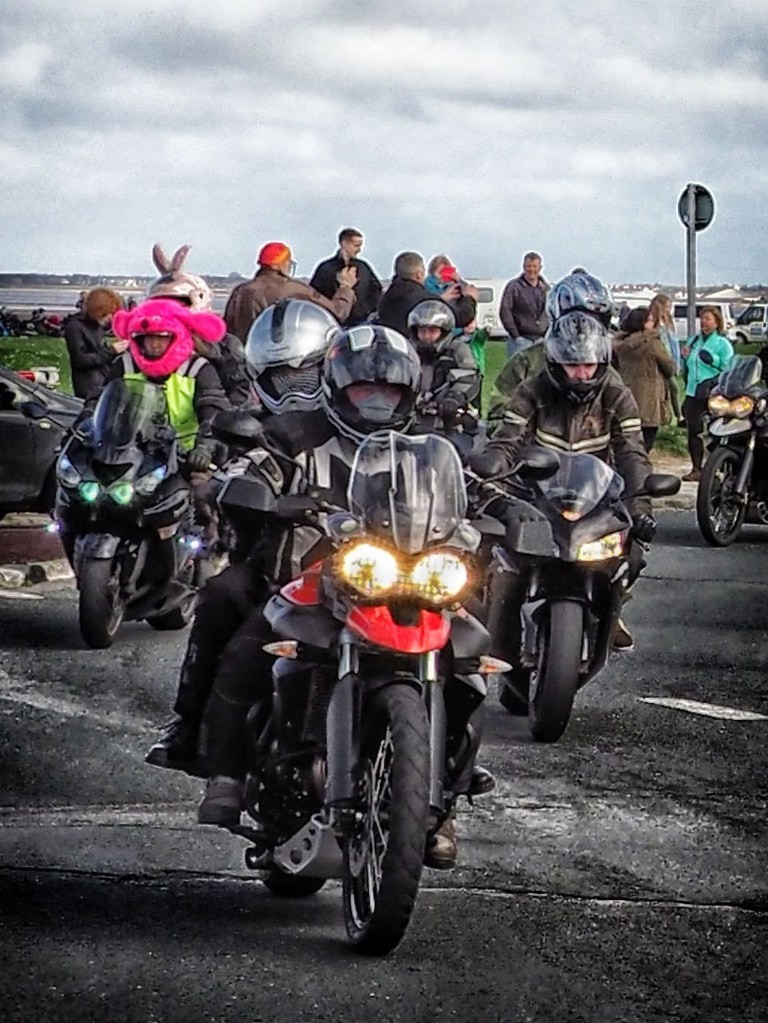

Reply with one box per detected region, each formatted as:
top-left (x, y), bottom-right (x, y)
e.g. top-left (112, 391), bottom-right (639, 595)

top-left (463, 764), bottom-right (495, 793)
top-left (424, 809), bottom-right (458, 869)
top-left (146, 717), bottom-right (200, 769)
top-left (198, 778), bottom-right (241, 824)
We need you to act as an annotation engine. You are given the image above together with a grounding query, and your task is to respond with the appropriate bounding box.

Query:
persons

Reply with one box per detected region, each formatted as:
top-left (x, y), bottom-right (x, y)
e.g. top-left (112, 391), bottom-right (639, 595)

top-left (61, 229), bottom-right (768, 588)
top-left (0, 306), bottom-right (66, 338)
top-left (473, 312), bottom-right (635, 677)
top-left (145, 300), bottom-right (493, 870)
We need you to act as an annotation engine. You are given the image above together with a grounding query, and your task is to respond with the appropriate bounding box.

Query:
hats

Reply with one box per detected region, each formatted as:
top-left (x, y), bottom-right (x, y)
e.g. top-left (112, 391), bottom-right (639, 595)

top-left (113, 297), bottom-right (226, 377)
top-left (258, 243), bottom-right (289, 265)
top-left (148, 242), bottom-right (211, 312)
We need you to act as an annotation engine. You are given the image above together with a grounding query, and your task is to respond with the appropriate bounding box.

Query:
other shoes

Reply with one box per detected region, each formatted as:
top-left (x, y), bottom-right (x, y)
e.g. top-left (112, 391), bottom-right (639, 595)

top-left (682, 467), bottom-right (704, 481)
top-left (610, 620), bottom-right (635, 649)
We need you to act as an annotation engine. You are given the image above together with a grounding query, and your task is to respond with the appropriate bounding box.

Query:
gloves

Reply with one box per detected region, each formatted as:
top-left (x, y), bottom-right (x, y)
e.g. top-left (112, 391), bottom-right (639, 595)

top-left (500, 504), bottom-right (548, 524)
top-left (186, 449), bottom-right (211, 472)
top-left (632, 513), bottom-right (657, 540)
top-left (698, 349), bottom-right (713, 364)
top-left (437, 398), bottom-right (460, 418)
top-left (73, 411), bottom-right (93, 433)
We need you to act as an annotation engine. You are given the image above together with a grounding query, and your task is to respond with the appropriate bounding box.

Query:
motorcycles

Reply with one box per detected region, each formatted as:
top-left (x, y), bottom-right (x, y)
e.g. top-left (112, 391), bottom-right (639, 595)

top-left (19, 376), bottom-right (241, 650)
top-left (207, 409), bottom-right (564, 960)
top-left (691, 349), bottom-right (768, 547)
top-left (404, 365), bottom-right (481, 481)
top-left (428, 431), bottom-right (682, 740)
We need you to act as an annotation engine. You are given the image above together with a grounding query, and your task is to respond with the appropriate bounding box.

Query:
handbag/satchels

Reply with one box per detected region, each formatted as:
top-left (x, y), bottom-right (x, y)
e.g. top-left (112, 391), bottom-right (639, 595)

top-left (683, 335), bottom-right (701, 383)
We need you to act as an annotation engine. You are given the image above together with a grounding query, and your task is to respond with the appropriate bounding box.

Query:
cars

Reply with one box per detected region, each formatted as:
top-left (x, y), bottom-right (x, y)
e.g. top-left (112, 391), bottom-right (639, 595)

top-left (606, 291), bottom-right (768, 350)
top-left (0, 368), bottom-right (88, 528)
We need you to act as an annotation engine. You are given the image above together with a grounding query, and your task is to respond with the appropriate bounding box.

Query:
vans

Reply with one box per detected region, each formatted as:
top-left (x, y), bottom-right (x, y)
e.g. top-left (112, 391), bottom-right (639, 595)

top-left (464, 279), bottom-right (512, 341)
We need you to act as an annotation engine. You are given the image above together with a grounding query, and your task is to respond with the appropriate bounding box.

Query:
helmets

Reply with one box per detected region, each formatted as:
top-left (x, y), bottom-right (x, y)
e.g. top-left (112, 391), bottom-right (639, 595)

top-left (408, 299), bottom-right (456, 360)
top-left (545, 273), bottom-right (612, 329)
top-left (131, 330), bottom-right (174, 360)
top-left (543, 312), bottom-right (612, 403)
top-left (322, 325), bottom-right (424, 448)
top-left (245, 297), bottom-right (344, 413)
